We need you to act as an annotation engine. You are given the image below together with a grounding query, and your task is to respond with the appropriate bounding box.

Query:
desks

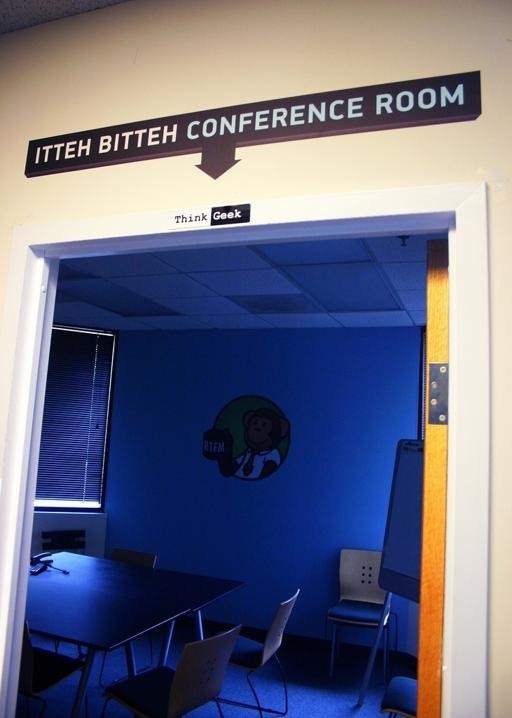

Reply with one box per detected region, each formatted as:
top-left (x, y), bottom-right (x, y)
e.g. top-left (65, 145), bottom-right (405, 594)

top-left (24, 574), bottom-right (192, 717)
top-left (22, 550), bottom-right (245, 716)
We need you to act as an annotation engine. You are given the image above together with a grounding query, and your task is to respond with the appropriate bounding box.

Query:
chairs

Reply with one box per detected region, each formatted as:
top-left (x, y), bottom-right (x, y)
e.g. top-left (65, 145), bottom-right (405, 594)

top-left (100, 622), bottom-right (242, 717)
top-left (41, 528), bottom-right (86, 556)
top-left (17, 618), bottom-right (86, 718)
top-left (214, 586), bottom-right (301, 718)
top-left (96, 548), bottom-right (161, 683)
top-left (326, 544), bottom-right (399, 686)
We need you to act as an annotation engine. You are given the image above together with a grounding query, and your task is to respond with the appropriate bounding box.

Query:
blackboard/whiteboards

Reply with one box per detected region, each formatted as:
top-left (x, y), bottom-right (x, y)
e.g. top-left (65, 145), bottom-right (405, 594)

top-left (376, 438), bottom-right (424, 605)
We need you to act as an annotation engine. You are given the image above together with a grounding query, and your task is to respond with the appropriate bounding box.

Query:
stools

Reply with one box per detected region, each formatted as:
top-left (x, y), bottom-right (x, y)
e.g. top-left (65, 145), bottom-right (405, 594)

top-left (379, 677), bottom-right (417, 718)
